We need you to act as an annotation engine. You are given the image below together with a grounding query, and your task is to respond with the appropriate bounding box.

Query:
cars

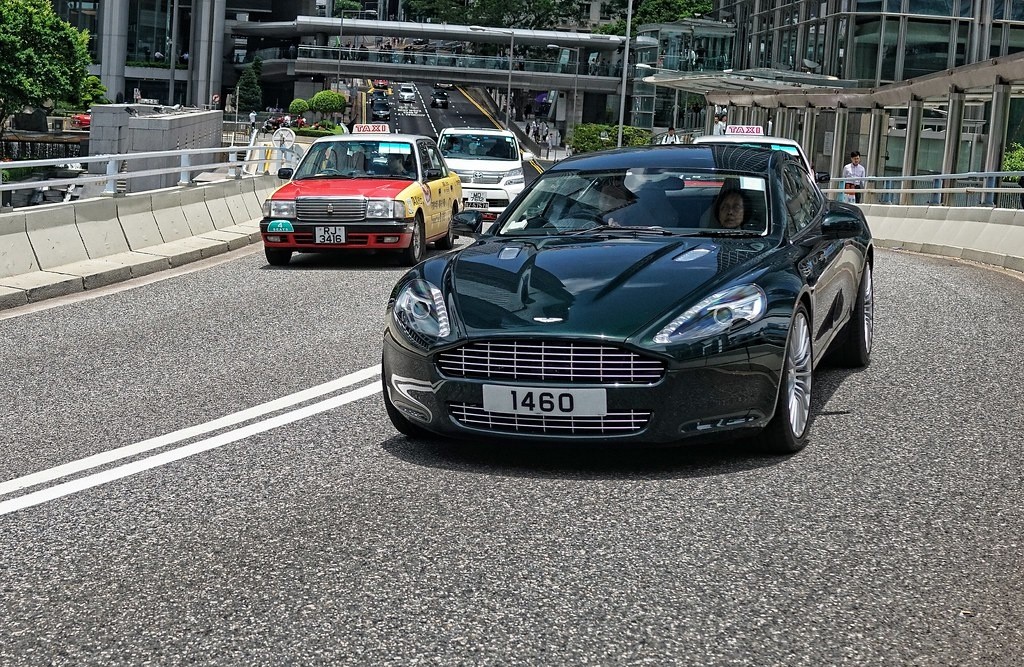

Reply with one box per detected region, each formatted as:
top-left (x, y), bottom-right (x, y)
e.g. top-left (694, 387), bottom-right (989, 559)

top-left (431, 83), bottom-right (454, 90)
top-left (397, 86), bottom-right (417, 102)
top-left (260, 125), bottom-right (463, 266)
top-left (412, 38), bottom-right (430, 45)
top-left (70, 109), bottom-right (93, 131)
top-left (678, 124), bottom-right (831, 192)
top-left (430, 88), bottom-right (450, 109)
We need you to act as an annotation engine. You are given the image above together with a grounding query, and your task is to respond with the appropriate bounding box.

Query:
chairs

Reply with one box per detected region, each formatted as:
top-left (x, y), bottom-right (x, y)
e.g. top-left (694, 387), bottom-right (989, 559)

top-left (699, 189), bottom-right (768, 232)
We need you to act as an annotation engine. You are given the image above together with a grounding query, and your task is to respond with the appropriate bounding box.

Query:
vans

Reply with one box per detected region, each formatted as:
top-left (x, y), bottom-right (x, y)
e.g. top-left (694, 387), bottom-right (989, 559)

top-left (428, 128), bottom-right (535, 223)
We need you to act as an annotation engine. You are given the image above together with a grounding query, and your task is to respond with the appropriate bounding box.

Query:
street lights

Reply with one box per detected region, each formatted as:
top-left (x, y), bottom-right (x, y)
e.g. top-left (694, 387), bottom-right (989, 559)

top-left (471, 25), bottom-right (514, 130)
top-left (363, 1), bottom-right (383, 43)
top-left (333, 9), bottom-right (377, 124)
top-left (547, 44), bottom-right (579, 146)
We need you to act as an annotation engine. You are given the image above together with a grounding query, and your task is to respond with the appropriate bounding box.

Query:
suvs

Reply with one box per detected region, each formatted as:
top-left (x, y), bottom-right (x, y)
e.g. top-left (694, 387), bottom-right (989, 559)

top-left (369, 91), bottom-right (389, 107)
top-left (371, 100), bottom-right (392, 121)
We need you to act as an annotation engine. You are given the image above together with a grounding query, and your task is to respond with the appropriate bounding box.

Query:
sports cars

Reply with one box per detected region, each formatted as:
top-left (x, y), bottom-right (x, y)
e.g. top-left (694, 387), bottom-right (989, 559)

top-left (382, 144), bottom-right (875, 456)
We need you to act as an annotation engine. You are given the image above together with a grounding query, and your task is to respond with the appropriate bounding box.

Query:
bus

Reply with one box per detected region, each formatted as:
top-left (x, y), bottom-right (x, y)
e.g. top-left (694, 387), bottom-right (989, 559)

top-left (372, 79), bottom-right (388, 97)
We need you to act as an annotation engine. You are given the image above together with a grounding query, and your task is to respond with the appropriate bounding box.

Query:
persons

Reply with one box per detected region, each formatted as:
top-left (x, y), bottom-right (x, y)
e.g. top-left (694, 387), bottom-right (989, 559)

top-left (493, 51), bottom-right (525, 70)
top-left (403, 45), bottom-right (429, 64)
top-left (331, 43), bottom-right (369, 61)
top-left (714, 187), bottom-right (753, 229)
top-left (289, 42), bottom-right (313, 57)
top-left (661, 127), bottom-right (681, 144)
top-left (714, 114), bottom-right (726, 134)
top-left (843, 151), bottom-right (866, 203)
top-left (597, 182), bottom-right (634, 227)
top-left (329, 144), bottom-right (353, 174)
top-left (590, 57), bottom-right (622, 77)
top-left (180, 51), bottom-right (189, 64)
top-left (767, 116), bottom-right (773, 135)
top-left (681, 44), bottom-right (707, 71)
top-left (379, 42), bottom-right (392, 62)
top-left (526, 118), bottom-right (553, 152)
top-left (659, 49), bottom-right (665, 69)
top-left (155, 50), bottom-right (164, 61)
top-left (719, 52), bottom-right (727, 70)
top-left (264, 106), bottom-right (319, 129)
top-left (386, 154), bottom-right (408, 175)
top-left (249, 110), bottom-right (257, 128)
